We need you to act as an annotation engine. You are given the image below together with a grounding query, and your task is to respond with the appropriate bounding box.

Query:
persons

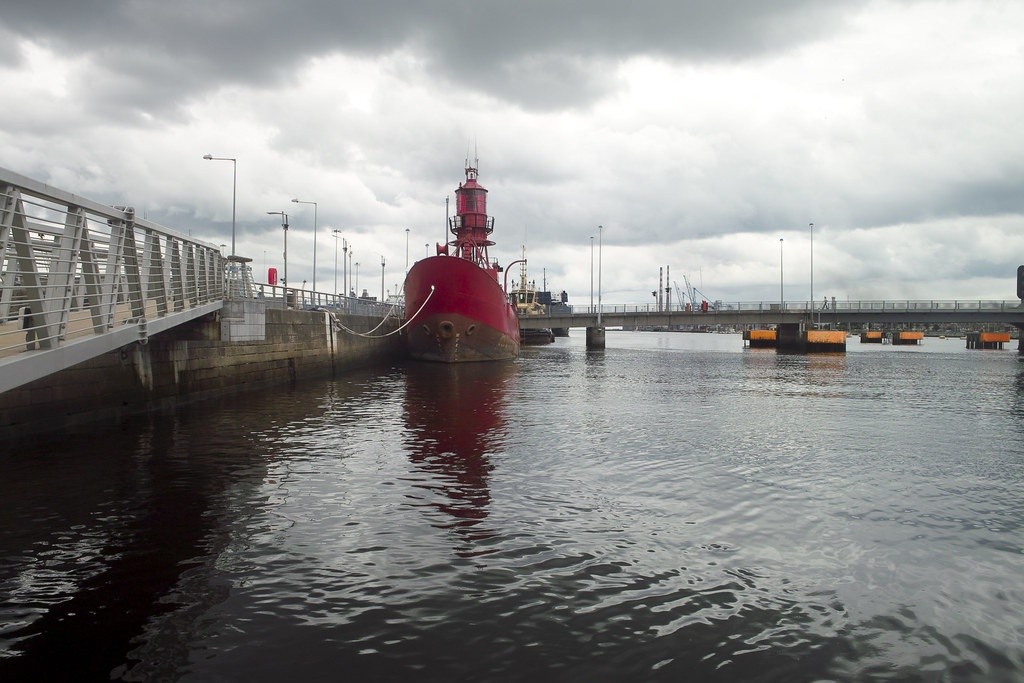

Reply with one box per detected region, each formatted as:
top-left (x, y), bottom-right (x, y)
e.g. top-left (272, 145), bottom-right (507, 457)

top-left (822, 297), bottom-right (829, 309)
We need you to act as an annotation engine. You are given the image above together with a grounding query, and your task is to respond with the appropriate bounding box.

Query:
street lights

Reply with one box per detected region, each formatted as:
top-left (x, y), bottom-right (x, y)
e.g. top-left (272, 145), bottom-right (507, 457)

top-left (778, 238), bottom-right (784, 310)
top-left (596, 225), bottom-right (604, 325)
top-left (588, 235), bottom-right (594, 314)
top-left (202, 153), bottom-right (238, 258)
top-left (405, 228), bottom-right (409, 270)
top-left (424, 243), bottom-right (430, 260)
top-left (267, 212), bottom-right (288, 297)
top-left (808, 223), bottom-right (814, 314)
top-left (292, 197), bottom-right (317, 305)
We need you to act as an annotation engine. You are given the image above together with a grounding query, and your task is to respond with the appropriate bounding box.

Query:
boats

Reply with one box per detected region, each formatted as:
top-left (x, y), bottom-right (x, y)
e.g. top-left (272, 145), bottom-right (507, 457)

top-left (509, 244), bottom-right (574, 343)
top-left (398, 140), bottom-right (524, 363)
